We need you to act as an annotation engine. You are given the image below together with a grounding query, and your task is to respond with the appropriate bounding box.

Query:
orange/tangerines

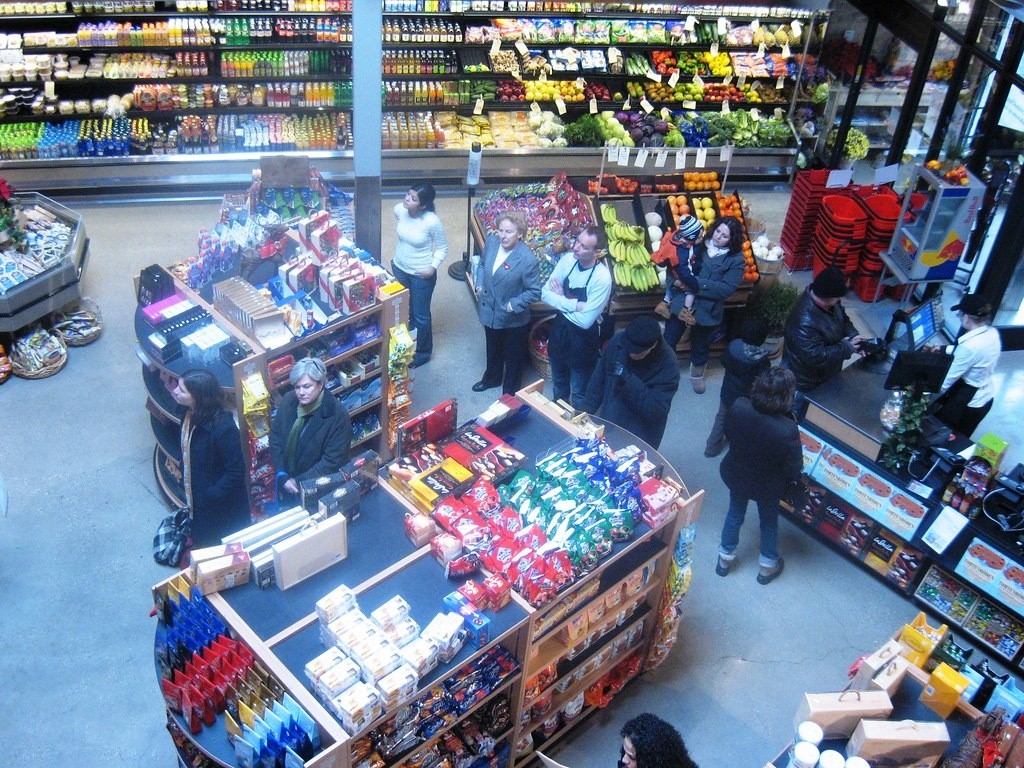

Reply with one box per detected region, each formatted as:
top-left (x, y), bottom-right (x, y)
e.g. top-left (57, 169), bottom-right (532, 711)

top-left (945, 166), bottom-right (965, 178)
top-left (683, 172), bottom-right (720, 190)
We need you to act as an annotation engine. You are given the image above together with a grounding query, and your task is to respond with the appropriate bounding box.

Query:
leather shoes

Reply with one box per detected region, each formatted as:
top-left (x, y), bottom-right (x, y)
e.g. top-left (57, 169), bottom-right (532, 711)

top-left (472, 381), bottom-right (501, 392)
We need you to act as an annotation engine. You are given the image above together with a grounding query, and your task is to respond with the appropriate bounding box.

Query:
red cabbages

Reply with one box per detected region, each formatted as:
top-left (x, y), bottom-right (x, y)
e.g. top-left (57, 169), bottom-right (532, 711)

top-left (617, 111), bottom-right (666, 147)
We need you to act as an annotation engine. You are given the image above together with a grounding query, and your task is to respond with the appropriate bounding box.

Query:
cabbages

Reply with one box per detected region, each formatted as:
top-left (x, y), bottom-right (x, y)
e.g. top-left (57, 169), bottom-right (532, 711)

top-left (594, 111), bottom-right (635, 146)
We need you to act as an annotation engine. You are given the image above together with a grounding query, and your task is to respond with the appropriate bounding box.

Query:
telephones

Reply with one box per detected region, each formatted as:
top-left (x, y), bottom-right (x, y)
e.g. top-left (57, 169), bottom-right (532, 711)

top-left (929, 446), bottom-right (966, 475)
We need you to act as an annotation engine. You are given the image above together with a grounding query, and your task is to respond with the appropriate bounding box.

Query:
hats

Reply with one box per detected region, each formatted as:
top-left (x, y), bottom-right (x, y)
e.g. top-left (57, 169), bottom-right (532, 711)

top-left (809, 266), bottom-right (849, 298)
top-left (677, 212), bottom-right (703, 241)
top-left (621, 316), bottom-right (661, 353)
top-left (950, 294), bottom-right (991, 317)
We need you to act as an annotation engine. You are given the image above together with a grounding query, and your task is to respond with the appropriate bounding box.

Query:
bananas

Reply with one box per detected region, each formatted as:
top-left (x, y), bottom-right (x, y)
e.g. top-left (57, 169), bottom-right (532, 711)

top-left (600, 203), bottom-right (660, 291)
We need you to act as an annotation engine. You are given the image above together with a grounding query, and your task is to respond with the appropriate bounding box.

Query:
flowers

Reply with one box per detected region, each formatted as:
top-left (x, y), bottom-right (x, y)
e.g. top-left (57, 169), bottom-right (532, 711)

top-left (826, 126), bottom-right (871, 161)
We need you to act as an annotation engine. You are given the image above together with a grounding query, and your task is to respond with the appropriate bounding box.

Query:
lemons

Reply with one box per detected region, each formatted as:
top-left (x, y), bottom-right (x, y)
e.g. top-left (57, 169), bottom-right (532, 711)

top-left (741, 84), bottom-right (762, 103)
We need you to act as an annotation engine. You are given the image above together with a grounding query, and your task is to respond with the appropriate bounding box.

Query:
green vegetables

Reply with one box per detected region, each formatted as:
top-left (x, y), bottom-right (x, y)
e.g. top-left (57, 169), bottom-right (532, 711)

top-left (811, 84), bottom-right (830, 104)
top-left (827, 127), bottom-right (869, 159)
top-left (562, 113), bottom-right (603, 147)
top-left (652, 106), bottom-right (789, 147)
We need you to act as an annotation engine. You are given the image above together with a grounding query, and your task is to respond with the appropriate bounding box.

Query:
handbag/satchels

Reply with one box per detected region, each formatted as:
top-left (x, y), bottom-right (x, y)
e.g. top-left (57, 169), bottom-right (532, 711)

top-left (153, 507), bottom-right (193, 571)
top-left (262, 471), bottom-right (301, 518)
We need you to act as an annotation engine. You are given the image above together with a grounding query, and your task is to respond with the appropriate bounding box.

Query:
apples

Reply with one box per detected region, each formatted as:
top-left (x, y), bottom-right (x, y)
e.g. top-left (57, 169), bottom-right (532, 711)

top-left (675, 82), bottom-right (745, 102)
top-left (498, 80), bottom-right (645, 102)
top-left (716, 191), bottom-right (759, 280)
top-left (586, 174), bottom-right (678, 194)
top-left (666, 196), bottom-right (690, 229)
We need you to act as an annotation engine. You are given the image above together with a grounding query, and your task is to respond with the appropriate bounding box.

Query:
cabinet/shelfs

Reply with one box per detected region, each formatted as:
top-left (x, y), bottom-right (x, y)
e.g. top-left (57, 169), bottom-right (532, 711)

top-left (347, 616), bottom-right (534, 768)
top-left (887, 162), bottom-right (988, 280)
top-left (133, 266), bottom-right (265, 511)
top-left (836, 86), bottom-right (935, 155)
top-left (0, 14), bottom-right (831, 209)
top-left (282, 215), bottom-right (410, 463)
top-left (514, 512), bottom-right (677, 768)
top-left (149, 565), bottom-right (348, 768)
top-left (265, 303), bottom-right (386, 467)
top-left (515, 379), bottom-right (706, 678)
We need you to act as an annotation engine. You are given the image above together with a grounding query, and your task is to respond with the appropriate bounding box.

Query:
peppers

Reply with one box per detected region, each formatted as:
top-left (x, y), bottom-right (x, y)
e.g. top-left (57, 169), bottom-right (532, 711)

top-left (652, 50), bottom-right (733, 77)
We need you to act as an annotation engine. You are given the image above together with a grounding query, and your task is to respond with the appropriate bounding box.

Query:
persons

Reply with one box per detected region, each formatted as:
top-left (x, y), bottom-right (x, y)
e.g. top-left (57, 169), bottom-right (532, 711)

top-left (704, 323), bottom-right (804, 585)
top-left (617, 712), bottom-right (700, 768)
top-left (930, 294), bottom-right (1001, 440)
top-left (173, 368), bottom-right (251, 548)
top-left (578, 316), bottom-right (681, 450)
top-left (268, 356), bottom-right (352, 514)
top-left (472, 211), bottom-right (542, 397)
top-left (780, 267), bottom-right (875, 425)
top-left (541, 226), bottom-right (612, 415)
top-left (391, 182), bottom-right (447, 369)
top-left (654, 215), bottom-right (745, 394)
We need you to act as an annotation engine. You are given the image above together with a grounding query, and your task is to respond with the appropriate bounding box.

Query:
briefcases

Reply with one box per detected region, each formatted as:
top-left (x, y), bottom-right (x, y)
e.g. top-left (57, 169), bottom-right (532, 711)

top-left (794, 638), bottom-right (951, 768)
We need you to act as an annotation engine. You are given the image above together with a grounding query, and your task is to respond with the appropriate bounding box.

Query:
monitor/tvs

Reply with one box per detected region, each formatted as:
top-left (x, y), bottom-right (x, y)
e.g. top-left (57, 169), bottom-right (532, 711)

top-left (884, 351), bottom-right (954, 393)
top-left (906, 299), bottom-right (937, 350)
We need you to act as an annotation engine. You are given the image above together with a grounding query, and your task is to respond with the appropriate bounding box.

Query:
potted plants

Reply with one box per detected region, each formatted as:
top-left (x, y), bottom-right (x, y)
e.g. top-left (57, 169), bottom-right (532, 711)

top-left (745, 277), bottom-right (801, 361)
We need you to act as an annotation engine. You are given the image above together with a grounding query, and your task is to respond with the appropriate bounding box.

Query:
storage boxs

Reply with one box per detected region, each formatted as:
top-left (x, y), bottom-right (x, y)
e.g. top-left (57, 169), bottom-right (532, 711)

top-left (973, 432), bottom-right (1010, 476)
top-left (137, 155), bottom-right (681, 739)
top-left (789, 563), bottom-right (1024, 768)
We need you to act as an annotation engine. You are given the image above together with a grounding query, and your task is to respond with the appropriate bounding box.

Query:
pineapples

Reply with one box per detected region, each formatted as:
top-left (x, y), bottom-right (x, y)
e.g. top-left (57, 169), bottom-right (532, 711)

top-left (753, 24), bottom-right (800, 45)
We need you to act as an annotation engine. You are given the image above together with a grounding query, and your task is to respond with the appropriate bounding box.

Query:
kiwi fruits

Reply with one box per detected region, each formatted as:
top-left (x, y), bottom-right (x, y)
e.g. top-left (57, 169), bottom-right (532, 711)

top-left (758, 84), bottom-right (787, 102)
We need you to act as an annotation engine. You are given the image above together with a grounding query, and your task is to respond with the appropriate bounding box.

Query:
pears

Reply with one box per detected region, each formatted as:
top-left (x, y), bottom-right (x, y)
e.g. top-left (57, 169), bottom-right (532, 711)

top-left (643, 81), bottom-right (676, 103)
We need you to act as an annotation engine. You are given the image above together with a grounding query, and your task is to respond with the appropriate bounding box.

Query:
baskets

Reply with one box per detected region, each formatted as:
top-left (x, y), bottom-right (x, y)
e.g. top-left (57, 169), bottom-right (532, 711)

top-left (527, 313), bottom-right (601, 382)
top-left (50, 304), bottom-right (104, 346)
top-left (8, 327), bottom-right (68, 379)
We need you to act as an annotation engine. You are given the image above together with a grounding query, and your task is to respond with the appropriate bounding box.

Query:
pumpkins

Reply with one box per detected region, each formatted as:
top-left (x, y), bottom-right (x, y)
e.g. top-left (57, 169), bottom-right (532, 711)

top-left (645, 212), bottom-right (666, 286)
top-left (752, 234), bottom-right (784, 261)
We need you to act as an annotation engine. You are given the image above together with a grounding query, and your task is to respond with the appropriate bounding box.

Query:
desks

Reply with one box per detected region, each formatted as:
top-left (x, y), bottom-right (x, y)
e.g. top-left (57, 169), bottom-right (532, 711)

top-left (870, 252), bottom-right (956, 340)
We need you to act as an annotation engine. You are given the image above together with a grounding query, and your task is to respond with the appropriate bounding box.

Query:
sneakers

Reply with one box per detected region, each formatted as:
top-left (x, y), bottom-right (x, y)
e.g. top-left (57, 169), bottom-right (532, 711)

top-left (654, 301), bottom-right (673, 319)
top-left (678, 307), bottom-right (696, 325)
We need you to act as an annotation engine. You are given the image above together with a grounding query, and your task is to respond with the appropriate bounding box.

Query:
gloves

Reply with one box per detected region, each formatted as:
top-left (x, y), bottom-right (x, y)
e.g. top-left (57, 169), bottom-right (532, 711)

top-left (693, 282), bottom-right (700, 294)
top-left (606, 358), bottom-right (631, 381)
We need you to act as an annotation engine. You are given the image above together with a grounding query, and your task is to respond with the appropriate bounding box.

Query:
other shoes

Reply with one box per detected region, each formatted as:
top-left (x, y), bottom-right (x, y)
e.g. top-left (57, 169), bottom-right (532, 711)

top-left (407, 358), bottom-right (430, 368)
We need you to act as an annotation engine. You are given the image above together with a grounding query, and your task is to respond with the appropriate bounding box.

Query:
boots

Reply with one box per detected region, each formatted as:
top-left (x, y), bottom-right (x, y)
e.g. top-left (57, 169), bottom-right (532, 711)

top-left (716, 552), bottom-right (736, 577)
top-left (756, 557), bottom-right (784, 585)
top-left (690, 362), bottom-right (707, 394)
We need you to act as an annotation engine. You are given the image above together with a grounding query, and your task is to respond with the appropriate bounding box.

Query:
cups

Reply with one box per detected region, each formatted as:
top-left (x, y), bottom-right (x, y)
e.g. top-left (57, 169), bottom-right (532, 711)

top-left (0, 54), bottom-right (69, 81)
top-left (67, 56), bottom-right (81, 66)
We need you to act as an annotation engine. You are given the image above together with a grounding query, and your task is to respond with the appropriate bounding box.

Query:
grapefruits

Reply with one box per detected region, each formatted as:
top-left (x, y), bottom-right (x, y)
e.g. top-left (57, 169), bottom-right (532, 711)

top-left (692, 196), bottom-right (716, 230)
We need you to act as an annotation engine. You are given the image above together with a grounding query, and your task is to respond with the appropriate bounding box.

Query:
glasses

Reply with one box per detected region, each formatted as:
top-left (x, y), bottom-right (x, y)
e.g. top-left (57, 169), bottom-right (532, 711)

top-left (496, 229), bottom-right (518, 235)
top-left (956, 312), bottom-right (964, 317)
top-left (294, 360), bottom-right (323, 374)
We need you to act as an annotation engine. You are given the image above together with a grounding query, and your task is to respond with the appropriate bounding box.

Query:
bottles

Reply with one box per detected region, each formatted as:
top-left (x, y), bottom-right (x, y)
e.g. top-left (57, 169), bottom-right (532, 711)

top-left (218, 81), bottom-right (352, 106)
top-left (71, 0), bottom-right (352, 13)
top-left (381, 50), bottom-right (457, 73)
top-left (382, 111), bottom-right (445, 149)
top-left (176, 52), bottom-right (207, 76)
top-left (273, 18), bottom-right (316, 42)
top-left (316, 17), bottom-right (353, 42)
top-left (77, 21), bottom-right (131, 46)
top-left (220, 51), bottom-right (309, 77)
top-left (130, 22), bottom-right (168, 46)
top-left (310, 50), bottom-right (353, 76)
top-left (168, 18), bottom-right (211, 46)
top-left (382, 0), bottom-right (423, 13)
top-left (0, 115), bottom-right (220, 160)
top-left (209, 18), bottom-right (274, 45)
top-left (381, 17), bottom-right (462, 42)
top-left (217, 112), bottom-right (353, 154)
top-left (382, 81), bottom-right (443, 106)
top-left (786, 721), bottom-right (870, 768)
top-left (103, 53), bottom-right (176, 78)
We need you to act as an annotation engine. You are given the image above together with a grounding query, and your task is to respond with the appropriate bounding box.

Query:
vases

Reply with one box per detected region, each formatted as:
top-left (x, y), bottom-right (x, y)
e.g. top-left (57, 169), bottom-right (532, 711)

top-left (838, 157), bottom-right (857, 170)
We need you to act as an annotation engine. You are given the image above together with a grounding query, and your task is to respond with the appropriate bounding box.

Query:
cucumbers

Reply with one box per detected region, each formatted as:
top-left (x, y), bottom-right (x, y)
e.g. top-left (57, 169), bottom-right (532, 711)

top-left (697, 20), bottom-right (726, 45)
top-left (471, 80), bottom-right (497, 102)
